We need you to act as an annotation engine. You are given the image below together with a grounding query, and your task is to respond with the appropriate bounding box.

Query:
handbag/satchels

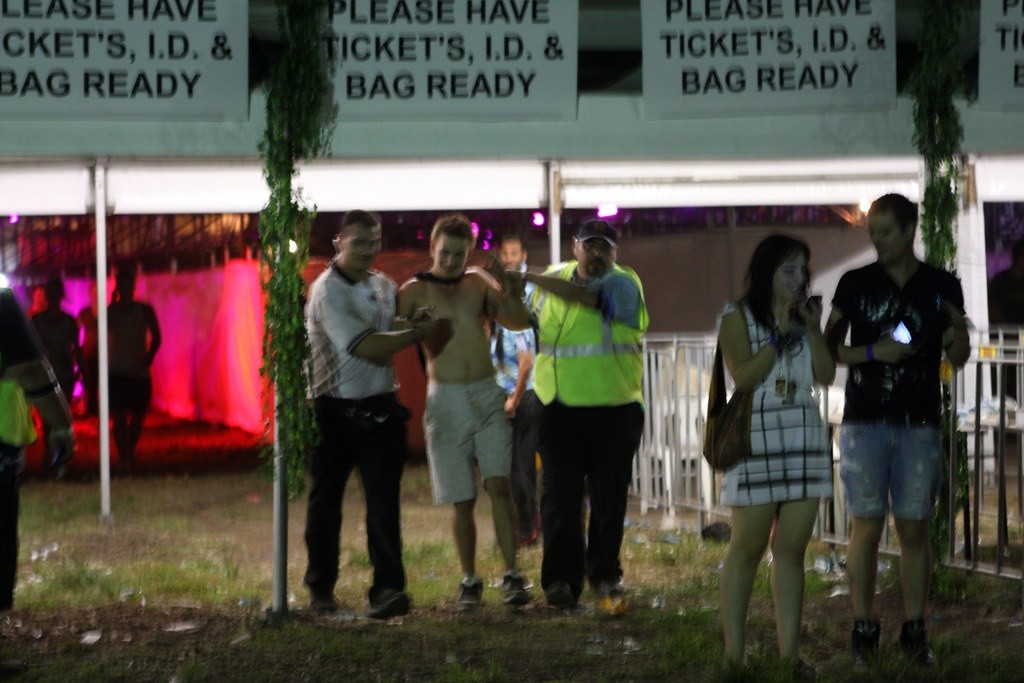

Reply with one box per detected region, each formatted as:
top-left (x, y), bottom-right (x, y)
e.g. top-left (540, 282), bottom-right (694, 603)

top-left (701, 304), bottom-right (753, 472)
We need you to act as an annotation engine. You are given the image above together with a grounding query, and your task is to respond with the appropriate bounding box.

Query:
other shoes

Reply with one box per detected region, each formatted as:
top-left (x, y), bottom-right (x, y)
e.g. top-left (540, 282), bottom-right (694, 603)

top-left (456, 576), bottom-right (484, 609)
top-left (597, 582), bottom-right (626, 603)
top-left (308, 592), bottom-right (342, 614)
top-left (849, 620), bottom-right (882, 673)
top-left (499, 573), bottom-right (530, 608)
top-left (370, 592), bottom-right (412, 619)
top-left (898, 619), bottom-right (936, 672)
top-left (552, 601), bottom-right (581, 618)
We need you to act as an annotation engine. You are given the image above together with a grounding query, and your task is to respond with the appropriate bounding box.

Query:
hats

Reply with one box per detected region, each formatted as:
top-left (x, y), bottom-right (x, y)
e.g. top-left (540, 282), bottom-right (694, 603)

top-left (576, 218), bottom-right (620, 248)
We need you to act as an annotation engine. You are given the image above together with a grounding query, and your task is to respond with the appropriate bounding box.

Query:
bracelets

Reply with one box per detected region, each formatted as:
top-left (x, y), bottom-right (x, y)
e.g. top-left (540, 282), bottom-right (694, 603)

top-left (865, 344), bottom-right (873, 362)
top-left (769, 337), bottom-right (777, 350)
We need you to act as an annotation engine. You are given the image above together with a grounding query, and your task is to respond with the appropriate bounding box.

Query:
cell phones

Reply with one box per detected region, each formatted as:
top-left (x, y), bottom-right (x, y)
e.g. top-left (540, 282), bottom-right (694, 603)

top-left (796, 294), bottom-right (822, 324)
top-left (892, 317), bottom-right (915, 345)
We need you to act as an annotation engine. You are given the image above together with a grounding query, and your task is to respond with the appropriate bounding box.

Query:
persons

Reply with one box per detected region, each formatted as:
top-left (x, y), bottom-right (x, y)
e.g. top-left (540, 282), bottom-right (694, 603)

top-left (0, 252), bottom-right (162, 616)
top-left (484, 233), bottom-right (539, 544)
top-left (303, 209), bottom-right (433, 619)
top-left (822, 194), bottom-right (970, 678)
top-left (487, 219), bottom-right (650, 618)
top-left (396, 215), bottom-right (534, 615)
top-left (719, 233), bottom-right (835, 683)
